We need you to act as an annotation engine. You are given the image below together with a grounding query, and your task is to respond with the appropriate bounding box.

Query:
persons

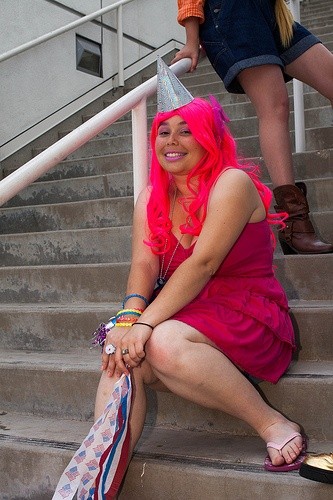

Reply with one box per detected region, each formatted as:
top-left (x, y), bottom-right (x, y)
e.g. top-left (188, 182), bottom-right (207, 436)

top-left (171, 0), bottom-right (333, 255)
top-left (89, 56), bottom-right (307, 472)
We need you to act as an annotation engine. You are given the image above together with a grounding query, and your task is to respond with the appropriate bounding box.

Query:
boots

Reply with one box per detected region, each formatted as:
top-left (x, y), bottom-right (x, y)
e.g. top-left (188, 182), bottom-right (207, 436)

top-left (273, 181), bottom-right (333, 254)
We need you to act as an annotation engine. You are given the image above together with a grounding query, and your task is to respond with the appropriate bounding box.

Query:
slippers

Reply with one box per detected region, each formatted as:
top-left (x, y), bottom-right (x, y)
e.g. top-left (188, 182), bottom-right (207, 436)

top-left (264, 432), bottom-right (306, 470)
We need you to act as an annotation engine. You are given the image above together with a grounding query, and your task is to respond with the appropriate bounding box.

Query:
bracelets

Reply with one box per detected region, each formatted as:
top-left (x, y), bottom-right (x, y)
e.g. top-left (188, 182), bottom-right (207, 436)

top-left (115, 295), bottom-right (148, 327)
top-left (131, 323), bottom-right (154, 330)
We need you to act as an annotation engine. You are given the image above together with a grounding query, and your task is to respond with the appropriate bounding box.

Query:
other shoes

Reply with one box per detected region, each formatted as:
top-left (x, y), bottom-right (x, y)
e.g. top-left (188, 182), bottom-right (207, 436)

top-left (299, 450), bottom-right (333, 484)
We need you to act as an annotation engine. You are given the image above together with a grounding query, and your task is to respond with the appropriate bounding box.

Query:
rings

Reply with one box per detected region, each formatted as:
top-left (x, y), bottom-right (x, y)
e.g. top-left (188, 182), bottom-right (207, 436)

top-left (121, 349), bottom-right (129, 354)
top-left (106, 344), bottom-right (116, 354)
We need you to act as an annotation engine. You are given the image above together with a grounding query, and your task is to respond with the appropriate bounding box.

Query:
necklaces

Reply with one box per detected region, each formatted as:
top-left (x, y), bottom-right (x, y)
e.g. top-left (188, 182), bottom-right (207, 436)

top-left (157, 186), bottom-right (190, 289)
